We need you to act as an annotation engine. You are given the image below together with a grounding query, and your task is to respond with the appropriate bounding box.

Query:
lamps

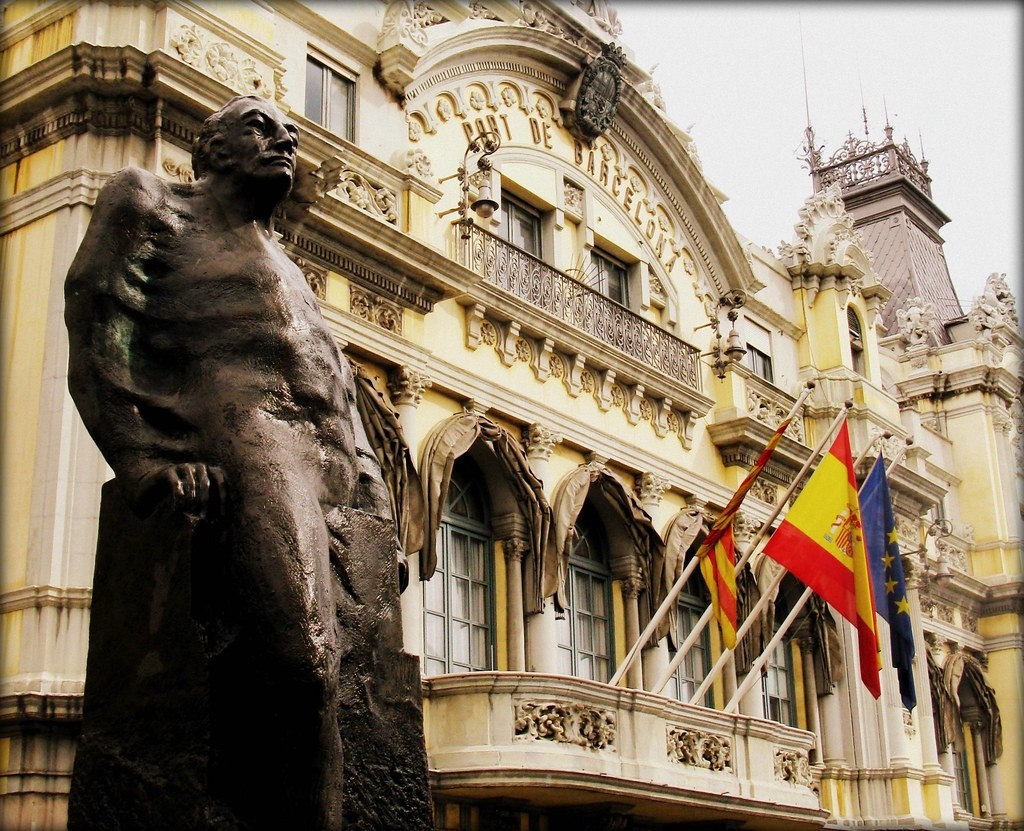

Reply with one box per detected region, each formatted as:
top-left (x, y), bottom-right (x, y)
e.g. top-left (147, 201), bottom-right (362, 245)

top-left (694, 286), bottom-right (752, 384)
top-left (442, 130), bottom-right (505, 228)
top-left (901, 516), bottom-right (959, 595)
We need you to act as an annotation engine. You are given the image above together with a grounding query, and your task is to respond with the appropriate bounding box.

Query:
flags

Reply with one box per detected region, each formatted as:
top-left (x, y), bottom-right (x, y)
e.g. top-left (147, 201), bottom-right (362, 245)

top-left (692, 416), bottom-right (917, 714)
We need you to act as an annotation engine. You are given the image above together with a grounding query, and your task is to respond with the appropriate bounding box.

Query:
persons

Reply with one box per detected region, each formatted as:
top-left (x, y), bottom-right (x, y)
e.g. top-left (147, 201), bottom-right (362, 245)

top-left (59, 95), bottom-right (412, 829)
